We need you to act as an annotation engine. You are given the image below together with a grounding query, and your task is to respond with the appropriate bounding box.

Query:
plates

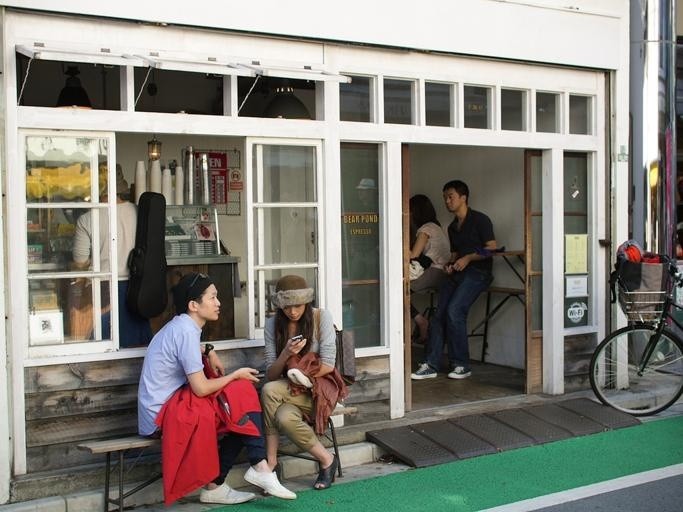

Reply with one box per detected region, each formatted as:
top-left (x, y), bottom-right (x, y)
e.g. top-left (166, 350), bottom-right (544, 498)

top-left (165, 242), bottom-right (215, 256)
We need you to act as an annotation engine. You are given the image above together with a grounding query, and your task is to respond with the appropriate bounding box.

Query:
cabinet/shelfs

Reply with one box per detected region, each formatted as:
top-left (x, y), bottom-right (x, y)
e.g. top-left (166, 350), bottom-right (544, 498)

top-left (26, 179), bottom-right (53, 269)
top-left (151, 201), bottom-right (220, 257)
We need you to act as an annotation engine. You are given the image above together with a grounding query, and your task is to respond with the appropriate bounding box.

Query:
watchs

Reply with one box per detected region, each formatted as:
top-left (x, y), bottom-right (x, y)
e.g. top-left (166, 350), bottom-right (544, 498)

top-left (204, 343), bottom-right (214, 355)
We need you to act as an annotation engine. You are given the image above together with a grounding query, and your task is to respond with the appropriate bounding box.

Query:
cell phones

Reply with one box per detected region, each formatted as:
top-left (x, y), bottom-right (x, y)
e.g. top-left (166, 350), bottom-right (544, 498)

top-left (292, 335), bottom-right (303, 343)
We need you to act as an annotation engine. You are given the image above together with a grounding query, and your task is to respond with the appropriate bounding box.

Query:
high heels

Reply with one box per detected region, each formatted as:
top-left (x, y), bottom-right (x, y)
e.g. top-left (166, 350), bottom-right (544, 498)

top-left (261, 462), bottom-right (281, 497)
top-left (312, 454), bottom-right (337, 490)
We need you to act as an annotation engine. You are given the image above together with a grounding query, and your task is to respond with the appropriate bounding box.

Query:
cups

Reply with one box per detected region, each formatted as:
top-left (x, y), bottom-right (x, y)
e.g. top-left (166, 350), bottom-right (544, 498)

top-left (183, 145), bottom-right (196, 205)
top-left (134, 158), bottom-right (184, 206)
top-left (197, 153), bottom-right (211, 205)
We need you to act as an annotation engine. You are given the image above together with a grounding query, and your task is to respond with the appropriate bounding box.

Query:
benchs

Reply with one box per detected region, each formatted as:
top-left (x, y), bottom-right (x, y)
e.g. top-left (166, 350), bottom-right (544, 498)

top-left (75, 405), bottom-right (359, 511)
top-left (464, 287), bottom-right (528, 372)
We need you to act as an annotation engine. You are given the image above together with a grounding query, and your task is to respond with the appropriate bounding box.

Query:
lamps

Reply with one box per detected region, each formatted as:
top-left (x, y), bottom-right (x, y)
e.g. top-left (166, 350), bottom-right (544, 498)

top-left (146, 132), bottom-right (164, 161)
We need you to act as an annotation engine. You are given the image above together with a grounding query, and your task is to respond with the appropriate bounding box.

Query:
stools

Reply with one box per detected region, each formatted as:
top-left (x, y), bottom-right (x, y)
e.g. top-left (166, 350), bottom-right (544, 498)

top-left (407, 283), bottom-right (446, 360)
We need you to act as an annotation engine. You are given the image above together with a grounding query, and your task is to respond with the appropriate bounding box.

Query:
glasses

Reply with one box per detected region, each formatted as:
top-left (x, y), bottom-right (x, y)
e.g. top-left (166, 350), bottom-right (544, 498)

top-left (190, 272), bottom-right (210, 288)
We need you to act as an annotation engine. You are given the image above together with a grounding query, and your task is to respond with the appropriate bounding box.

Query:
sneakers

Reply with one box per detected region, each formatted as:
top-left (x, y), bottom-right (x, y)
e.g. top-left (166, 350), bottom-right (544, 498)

top-left (447, 367), bottom-right (471, 379)
top-left (410, 363), bottom-right (437, 380)
top-left (199, 481), bottom-right (255, 505)
top-left (243, 466), bottom-right (297, 500)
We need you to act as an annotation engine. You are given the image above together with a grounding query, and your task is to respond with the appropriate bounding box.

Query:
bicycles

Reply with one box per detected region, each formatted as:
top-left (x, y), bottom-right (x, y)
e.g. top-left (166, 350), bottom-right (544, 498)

top-left (588, 240), bottom-right (683, 416)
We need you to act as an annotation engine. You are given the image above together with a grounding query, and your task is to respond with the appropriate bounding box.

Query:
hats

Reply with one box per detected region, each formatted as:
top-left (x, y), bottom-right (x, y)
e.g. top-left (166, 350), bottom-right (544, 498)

top-left (270, 275), bottom-right (314, 310)
top-left (99, 162), bottom-right (128, 195)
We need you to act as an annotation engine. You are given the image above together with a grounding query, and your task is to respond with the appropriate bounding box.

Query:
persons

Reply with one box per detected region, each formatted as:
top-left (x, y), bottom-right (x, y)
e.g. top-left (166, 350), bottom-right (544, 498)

top-left (136, 271), bottom-right (298, 506)
top-left (410, 180), bottom-right (497, 380)
top-left (408, 194), bottom-right (452, 341)
top-left (348, 177), bottom-right (378, 279)
top-left (69, 160), bottom-right (153, 349)
top-left (260, 274), bottom-right (354, 498)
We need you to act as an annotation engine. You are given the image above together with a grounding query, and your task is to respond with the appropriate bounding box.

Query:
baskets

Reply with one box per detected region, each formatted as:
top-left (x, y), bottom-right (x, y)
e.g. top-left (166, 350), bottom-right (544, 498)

top-left (617, 282), bottom-right (666, 322)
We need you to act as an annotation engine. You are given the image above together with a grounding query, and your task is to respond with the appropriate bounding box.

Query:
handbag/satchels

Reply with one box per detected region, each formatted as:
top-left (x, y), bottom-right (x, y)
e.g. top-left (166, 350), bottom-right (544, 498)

top-left (313, 308), bottom-right (356, 386)
top-left (409, 260), bottom-right (425, 281)
top-left (607, 252), bottom-right (669, 317)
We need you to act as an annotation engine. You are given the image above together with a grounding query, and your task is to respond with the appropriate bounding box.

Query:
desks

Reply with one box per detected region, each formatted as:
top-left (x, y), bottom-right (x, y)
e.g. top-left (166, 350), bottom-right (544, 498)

top-left (479, 243), bottom-right (527, 288)
